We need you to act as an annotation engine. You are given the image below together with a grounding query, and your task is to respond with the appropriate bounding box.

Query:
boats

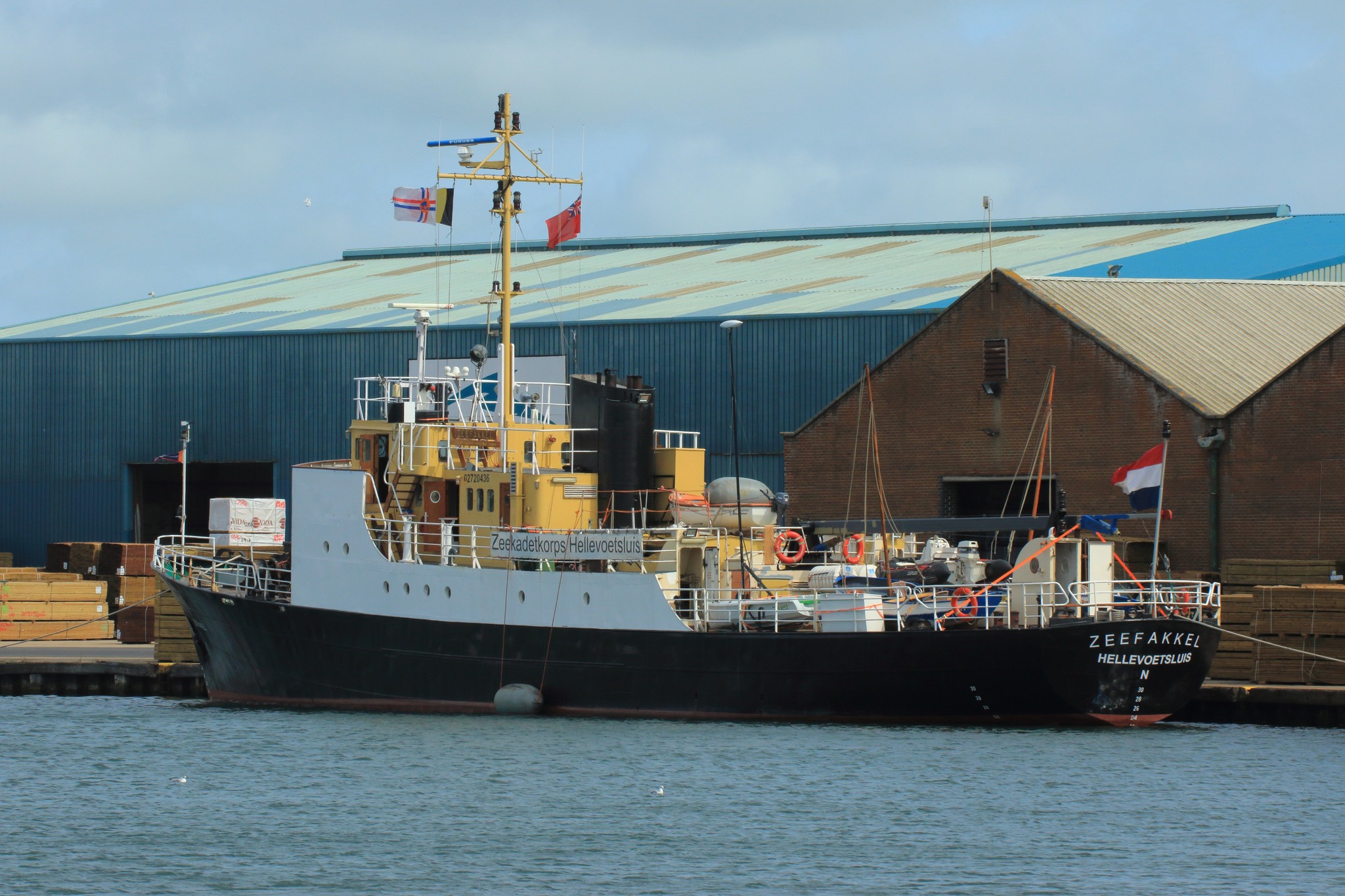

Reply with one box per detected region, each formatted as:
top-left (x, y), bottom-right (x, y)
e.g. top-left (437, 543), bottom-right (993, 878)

top-left (146, 88), bottom-right (1224, 734)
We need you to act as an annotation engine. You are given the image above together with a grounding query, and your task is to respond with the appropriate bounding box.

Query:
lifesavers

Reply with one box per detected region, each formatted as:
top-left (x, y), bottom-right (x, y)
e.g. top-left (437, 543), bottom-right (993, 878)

top-left (1173, 588), bottom-right (1189, 617)
top-left (951, 587), bottom-right (978, 622)
top-left (842, 534), bottom-right (864, 564)
top-left (774, 531), bottom-right (806, 564)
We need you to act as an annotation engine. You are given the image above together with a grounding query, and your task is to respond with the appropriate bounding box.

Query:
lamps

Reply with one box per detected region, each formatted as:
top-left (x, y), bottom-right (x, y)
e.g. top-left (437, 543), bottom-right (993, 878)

top-left (470, 344), bottom-right (488, 368)
top-left (683, 527), bottom-right (698, 538)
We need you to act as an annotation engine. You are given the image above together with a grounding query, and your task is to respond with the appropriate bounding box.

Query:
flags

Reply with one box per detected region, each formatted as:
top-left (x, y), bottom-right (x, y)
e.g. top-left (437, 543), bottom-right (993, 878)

top-left (545, 194), bottom-right (582, 250)
top-left (392, 186), bottom-right (455, 226)
top-left (153, 450), bottom-right (185, 463)
top-left (1111, 442), bottom-right (1166, 511)
